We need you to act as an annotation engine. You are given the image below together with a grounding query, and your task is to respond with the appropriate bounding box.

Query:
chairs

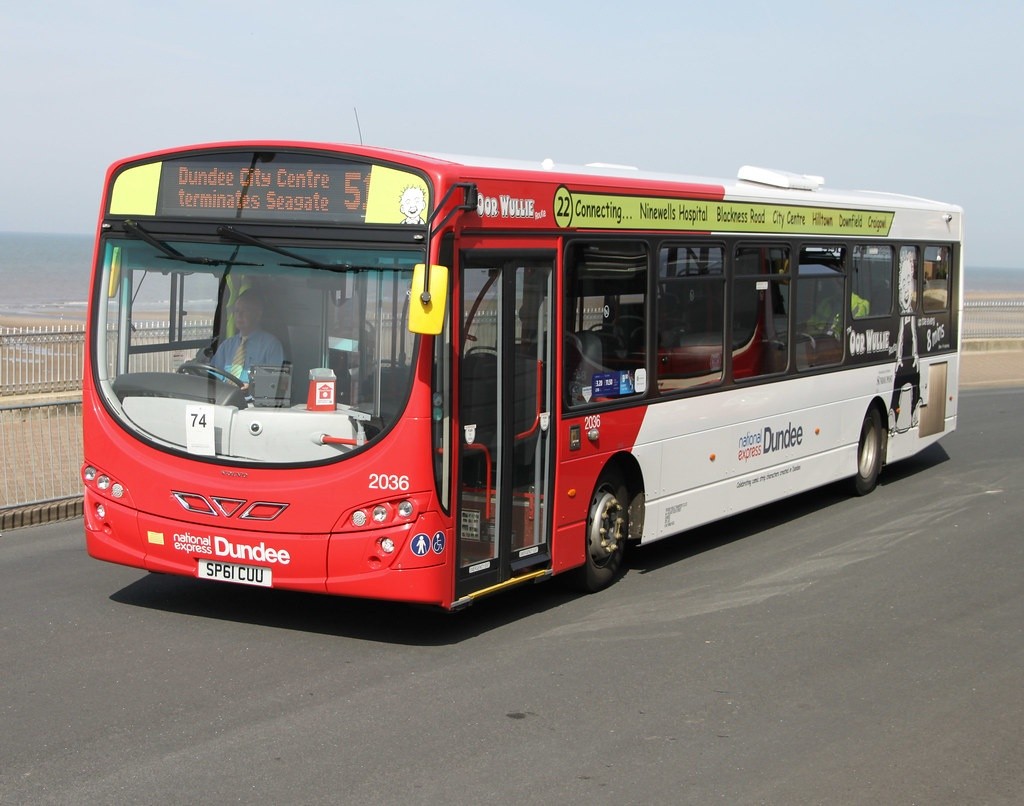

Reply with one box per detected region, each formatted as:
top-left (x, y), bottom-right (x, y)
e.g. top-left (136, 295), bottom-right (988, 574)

top-left (355, 312), bottom-right (841, 481)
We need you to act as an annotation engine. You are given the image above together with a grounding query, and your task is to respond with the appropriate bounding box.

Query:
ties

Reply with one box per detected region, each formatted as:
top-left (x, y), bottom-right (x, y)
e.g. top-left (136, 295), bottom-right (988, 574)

top-left (228, 336), bottom-right (248, 387)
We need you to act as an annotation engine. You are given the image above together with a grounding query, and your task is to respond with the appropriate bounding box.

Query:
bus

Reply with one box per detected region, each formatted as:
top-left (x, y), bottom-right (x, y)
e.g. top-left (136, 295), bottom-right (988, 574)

top-left (80, 137), bottom-right (965, 614)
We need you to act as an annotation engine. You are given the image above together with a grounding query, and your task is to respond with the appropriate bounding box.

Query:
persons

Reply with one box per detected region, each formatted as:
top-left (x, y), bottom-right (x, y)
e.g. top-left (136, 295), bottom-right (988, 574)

top-left (206, 294), bottom-right (284, 402)
top-left (804, 277), bottom-right (870, 341)
